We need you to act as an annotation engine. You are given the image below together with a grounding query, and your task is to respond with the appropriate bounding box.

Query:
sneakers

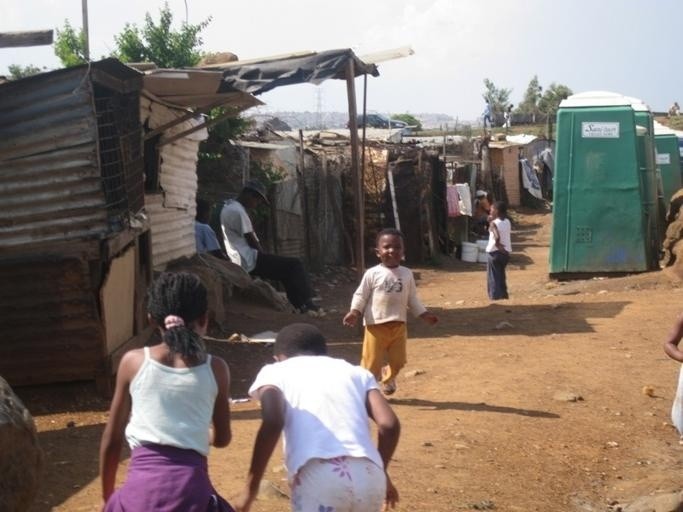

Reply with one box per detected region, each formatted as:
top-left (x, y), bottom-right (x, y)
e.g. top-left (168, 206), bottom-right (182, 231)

top-left (380, 366), bottom-right (396, 395)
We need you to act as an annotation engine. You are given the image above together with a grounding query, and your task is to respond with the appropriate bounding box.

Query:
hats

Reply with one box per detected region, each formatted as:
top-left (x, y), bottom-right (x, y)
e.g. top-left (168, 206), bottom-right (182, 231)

top-left (244, 177), bottom-right (270, 205)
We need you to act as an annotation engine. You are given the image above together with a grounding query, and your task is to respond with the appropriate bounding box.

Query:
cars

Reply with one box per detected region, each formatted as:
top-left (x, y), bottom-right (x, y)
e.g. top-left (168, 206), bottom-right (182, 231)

top-left (344, 111), bottom-right (408, 129)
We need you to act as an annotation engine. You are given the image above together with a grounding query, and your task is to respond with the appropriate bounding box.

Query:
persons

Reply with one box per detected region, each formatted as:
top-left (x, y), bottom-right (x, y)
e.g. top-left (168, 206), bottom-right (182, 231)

top-left (501, 104), bottom-right (514, 128)
top-left (220, 178), bottom-right (321, 314)
top-left (343, 226), bottom-right (439, 396)
top-left (481, 99), bottom-right (494, 129)
top-left (663, 313), bottom-right (683, 438)
top-left (233, 322), bottom-right (403, 511)
top-left (473, 190), bottom-right (499, 228)
top-left (666, 102), bottom-right (680, 120)
top-left (486, 202), bottom-right (515, 300)
top-left (194, 199), bottom-right (228, 261)
top-left (97, 271), bottom-right (236, 512)
